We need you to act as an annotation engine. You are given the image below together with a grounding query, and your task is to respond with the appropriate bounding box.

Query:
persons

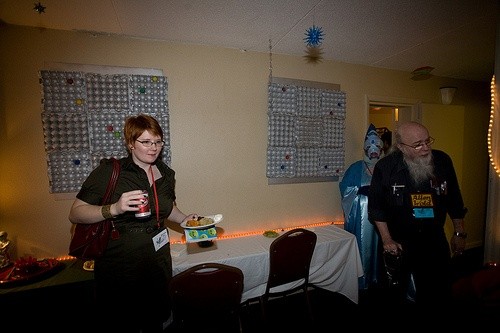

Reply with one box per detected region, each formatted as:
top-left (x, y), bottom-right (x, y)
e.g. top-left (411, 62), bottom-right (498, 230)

top-left (368, 121), bottom-right (467, 304)
top-left (68, 114), bottom-right (198, 333)
top-left (340, 123), bottom-right (384, 289)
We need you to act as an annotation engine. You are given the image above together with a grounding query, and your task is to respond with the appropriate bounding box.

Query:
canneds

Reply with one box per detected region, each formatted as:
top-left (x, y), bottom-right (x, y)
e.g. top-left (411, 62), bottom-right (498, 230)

top-left (133, 190), bottom-right (151, 217)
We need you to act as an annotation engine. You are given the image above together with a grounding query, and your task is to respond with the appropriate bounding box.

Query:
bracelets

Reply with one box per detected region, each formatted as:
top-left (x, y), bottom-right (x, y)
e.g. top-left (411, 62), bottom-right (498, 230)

top-left (453, 231), bottom-right (467, 238)
top-left (102, 204), bottom-right (117, 219)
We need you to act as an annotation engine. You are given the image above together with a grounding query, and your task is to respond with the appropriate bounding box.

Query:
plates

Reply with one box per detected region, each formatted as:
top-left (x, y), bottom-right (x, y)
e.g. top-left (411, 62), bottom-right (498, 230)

top-left (180, 214), bottom-right (222, 229)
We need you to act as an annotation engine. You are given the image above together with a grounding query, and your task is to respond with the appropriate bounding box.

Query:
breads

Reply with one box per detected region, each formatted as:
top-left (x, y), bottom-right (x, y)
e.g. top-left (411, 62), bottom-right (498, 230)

top-left (84, 260), bottom-right (94, 269)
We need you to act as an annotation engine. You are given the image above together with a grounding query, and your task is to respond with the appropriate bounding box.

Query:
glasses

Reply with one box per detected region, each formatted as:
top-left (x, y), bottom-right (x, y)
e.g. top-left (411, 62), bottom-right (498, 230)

top-left (400, 136), bottom-right (435, 151)
top-left (136, 139), bottom-right (165, 147)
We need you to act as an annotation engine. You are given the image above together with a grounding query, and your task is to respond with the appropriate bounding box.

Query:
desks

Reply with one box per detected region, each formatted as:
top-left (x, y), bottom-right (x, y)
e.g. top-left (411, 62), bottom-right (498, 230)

top-left (170, 224), bottom-right (364, 304)
top-left (0, 259), bottom-right (95, 333)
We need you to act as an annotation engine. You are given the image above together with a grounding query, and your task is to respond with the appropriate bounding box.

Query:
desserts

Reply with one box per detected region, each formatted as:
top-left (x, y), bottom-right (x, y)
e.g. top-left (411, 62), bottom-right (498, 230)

top-left (186, 215), bottom-right (213, 227)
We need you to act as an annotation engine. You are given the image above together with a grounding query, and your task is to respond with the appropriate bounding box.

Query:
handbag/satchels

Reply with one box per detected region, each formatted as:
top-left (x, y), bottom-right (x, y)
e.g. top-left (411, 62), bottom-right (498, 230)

top-left (68, 157), bottom-right (120, 258)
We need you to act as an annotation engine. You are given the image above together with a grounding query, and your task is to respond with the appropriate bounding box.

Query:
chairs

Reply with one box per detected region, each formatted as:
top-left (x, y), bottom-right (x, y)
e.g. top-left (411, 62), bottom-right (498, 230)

top-left (169, 263), bottom-right (244, 333)
top-left (255, 229), bottom-right (317, 326)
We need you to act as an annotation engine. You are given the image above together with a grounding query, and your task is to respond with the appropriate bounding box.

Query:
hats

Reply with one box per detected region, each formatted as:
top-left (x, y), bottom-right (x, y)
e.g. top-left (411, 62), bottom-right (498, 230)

top-left (364, 124), bottom-right (384, 151)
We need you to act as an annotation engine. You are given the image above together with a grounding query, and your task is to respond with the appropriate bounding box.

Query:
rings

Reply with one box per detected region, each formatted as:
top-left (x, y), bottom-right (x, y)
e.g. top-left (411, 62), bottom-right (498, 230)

top-left (383, 249), bottom-right (397, 254)
